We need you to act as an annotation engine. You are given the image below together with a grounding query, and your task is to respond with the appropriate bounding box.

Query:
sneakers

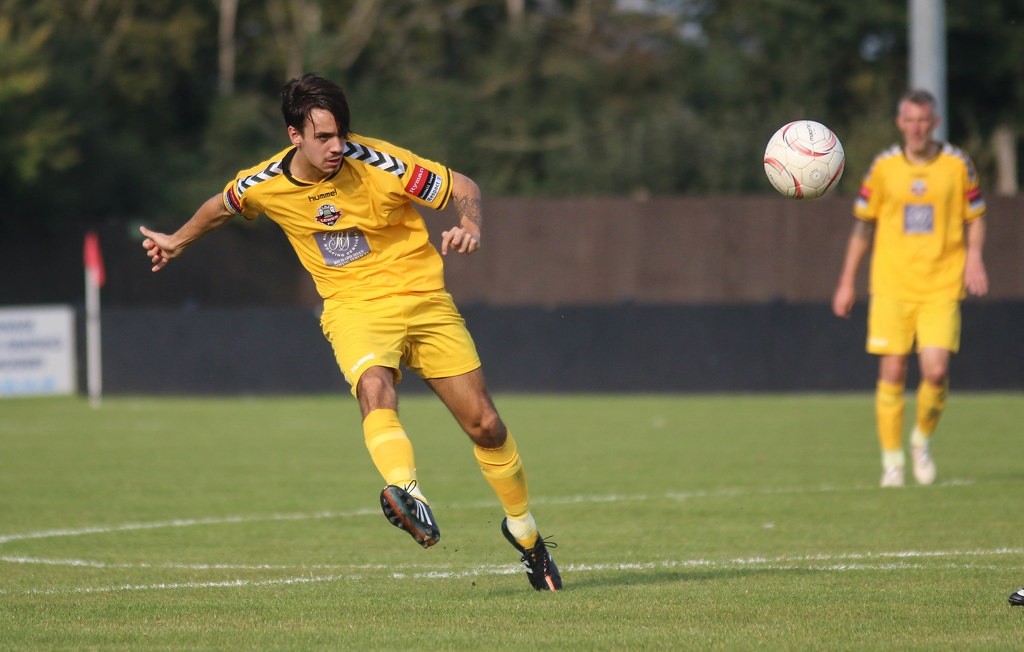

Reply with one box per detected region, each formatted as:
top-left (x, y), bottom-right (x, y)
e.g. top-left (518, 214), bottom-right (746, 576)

top-left (378, 479), bottom-right (442, 549)
top-left (910, 443), bottom-right (938, 486)
top-left (881, 465), bottom-right (904, 488)
top-left (499, 515), bottom-right (564, 591)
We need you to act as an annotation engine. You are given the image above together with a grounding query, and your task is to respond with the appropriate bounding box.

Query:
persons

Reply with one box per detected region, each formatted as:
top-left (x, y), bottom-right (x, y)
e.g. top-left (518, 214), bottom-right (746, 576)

top-left (831, 89), bottom-right (990, 487)
top-left (138, 72), bottom-right (563, 591)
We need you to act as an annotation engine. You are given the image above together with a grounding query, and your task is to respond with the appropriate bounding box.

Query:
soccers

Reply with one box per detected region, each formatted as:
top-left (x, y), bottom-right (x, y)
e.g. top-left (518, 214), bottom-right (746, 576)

top-left (763, 120), bottom-right (846, 200)
